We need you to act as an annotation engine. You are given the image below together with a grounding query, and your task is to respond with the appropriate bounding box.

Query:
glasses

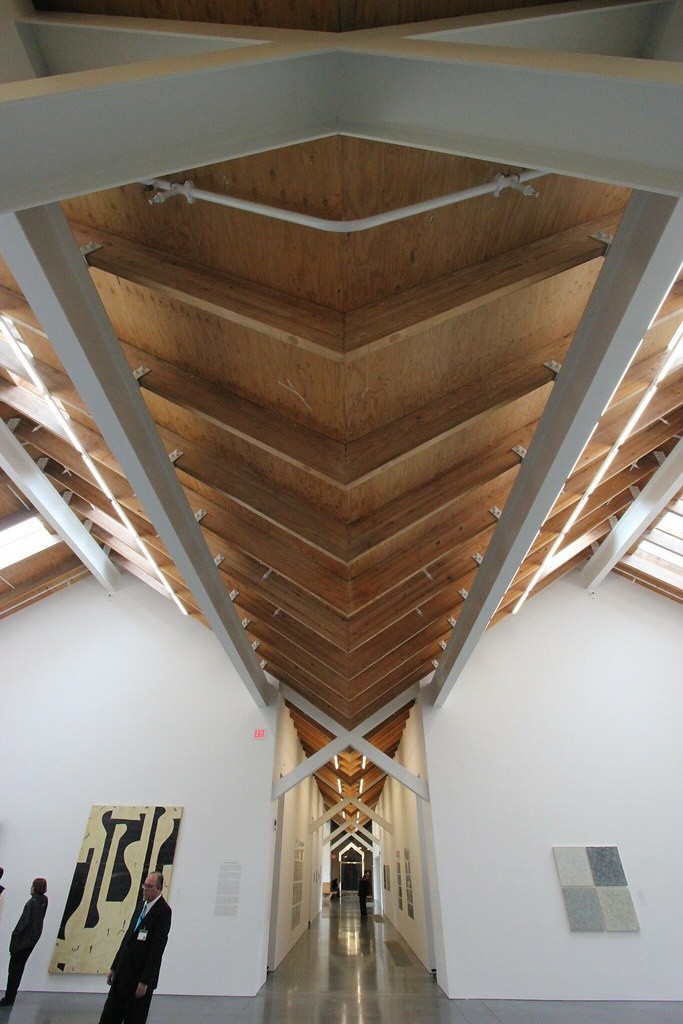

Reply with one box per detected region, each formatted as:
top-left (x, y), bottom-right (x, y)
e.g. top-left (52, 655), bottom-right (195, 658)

top-left (142, 884), bottom-right (157, 888)
top-left (31, 886), bottom-right (34, 887)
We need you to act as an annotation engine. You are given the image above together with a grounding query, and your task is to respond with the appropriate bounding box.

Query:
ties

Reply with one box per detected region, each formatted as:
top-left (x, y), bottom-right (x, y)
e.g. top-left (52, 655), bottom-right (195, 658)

top-left (133, 905), bottom-right (148, 932)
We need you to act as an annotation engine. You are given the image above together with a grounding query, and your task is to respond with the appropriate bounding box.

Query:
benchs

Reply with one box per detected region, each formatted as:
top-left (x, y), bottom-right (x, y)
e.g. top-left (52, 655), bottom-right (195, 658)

top-left (332, 891), bottom-right (338, 901)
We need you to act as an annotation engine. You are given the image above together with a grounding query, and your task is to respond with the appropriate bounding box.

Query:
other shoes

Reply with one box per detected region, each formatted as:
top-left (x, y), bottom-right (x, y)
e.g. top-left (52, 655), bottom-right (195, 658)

top-left (0, 998), bottom-right (15, 1007)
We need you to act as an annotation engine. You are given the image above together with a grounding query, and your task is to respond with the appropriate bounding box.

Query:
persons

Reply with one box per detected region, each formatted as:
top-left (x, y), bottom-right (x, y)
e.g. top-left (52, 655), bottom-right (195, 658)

top-left (0, 879), bottom-right (48, 1007)
top-left (357, 875), bottom-right (371, 916)
top-left (98, 871), bottom-right (172, 1024)
top-left (332, 878), bottom-right (340, 897)
